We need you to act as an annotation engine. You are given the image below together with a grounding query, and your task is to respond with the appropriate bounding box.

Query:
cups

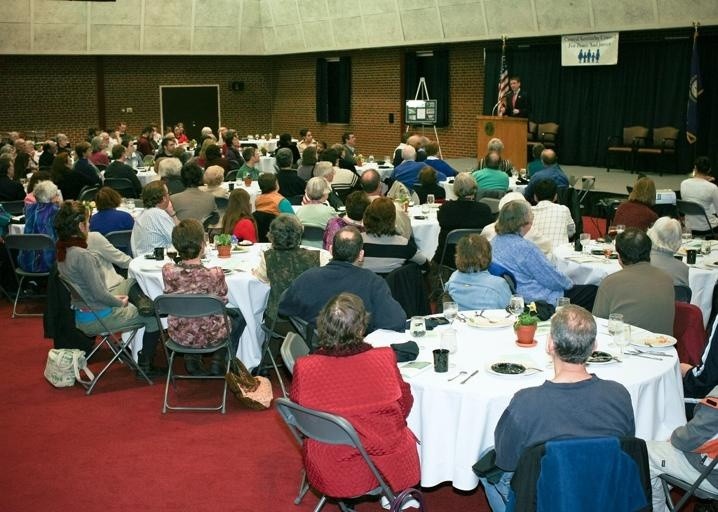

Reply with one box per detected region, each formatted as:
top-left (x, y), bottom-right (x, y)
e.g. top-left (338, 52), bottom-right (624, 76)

top-left (228, 177), bottom-right (251, 191)
top-left (154, 248), bottom-right (164, 260)
top-left (687, 249), bottom-right (696, 264)
top-left (120, 198), bottom-right (135, 214)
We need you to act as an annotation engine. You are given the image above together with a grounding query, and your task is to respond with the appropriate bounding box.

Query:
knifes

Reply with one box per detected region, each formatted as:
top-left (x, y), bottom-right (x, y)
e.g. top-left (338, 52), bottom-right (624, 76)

top-left (460, 368), bottom-right (478, 386)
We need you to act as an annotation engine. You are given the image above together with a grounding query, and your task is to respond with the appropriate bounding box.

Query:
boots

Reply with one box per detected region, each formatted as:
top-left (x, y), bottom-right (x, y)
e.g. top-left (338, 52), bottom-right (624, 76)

top-left (184, 353), bottom-right (210, 376)
top-left (209, 349), bottom-right (226, 376)
top-left (136, 350), bottom-right (168, 382)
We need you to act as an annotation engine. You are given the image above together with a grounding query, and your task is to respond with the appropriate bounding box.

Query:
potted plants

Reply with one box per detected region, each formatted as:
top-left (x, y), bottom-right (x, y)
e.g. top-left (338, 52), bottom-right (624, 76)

top-left (511, 308), bottom-right (541, 348)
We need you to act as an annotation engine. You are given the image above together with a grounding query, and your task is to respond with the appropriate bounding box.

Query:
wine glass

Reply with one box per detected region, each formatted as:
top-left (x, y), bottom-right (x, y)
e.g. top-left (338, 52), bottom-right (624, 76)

top-left (166, 247), bottom-right (177, 263)
top-left (427, 194), bottom-right (435, 205)
top-left (681, 226), bottom-right (692, 250)
top-left (422, 204), bottom-right (428, 221)
top-left (410, 294), bottom-right (631, 362)
top-left (511, 168), bottom-right (526, 180)
top-left (700, 241), bottom-right (711, 259)
top-left (255, 133), bottom-right (272, 141)
top-left (369, 156), bottom-right (374, 165)
top-left (579, 225), bottom-right (629, 257)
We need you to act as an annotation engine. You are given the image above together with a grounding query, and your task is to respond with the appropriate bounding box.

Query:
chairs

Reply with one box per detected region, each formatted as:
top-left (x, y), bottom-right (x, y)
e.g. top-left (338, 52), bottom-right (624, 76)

top-left (511, 433), bottom-right (653, 512)
top-left (657, 454), bottom-right (718, 512)
top-left (150, 293), bottom-right (241, 415)
top-left (279, 329), bottom-right (310, 374)
top-left (57, 273), bottom-right (153, 392)
top-left (253, 288), bottom-right (293, 400)
top-left (674, 299), bottom-right (707, 366)
top-left (274, 397), bottom-right (397, 511)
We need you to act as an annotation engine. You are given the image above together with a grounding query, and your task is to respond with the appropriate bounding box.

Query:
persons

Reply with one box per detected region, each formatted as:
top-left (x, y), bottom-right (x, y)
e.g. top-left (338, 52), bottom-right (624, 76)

top-left (277, 138), bottom-right (717, 512)
top-left (0, 122), bottom-right (458, 389)
top-left (499, 74), bottom-right (532, 117)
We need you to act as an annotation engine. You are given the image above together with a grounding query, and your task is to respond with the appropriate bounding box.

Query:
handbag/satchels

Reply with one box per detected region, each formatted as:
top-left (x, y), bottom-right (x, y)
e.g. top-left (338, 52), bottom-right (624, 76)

top-left (225, 355), bottom-right (274, 411)
top-left (41, 347), bottom-right (95, 388)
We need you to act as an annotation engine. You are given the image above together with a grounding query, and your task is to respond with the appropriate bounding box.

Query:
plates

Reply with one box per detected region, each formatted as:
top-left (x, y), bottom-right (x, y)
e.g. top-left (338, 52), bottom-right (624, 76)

top-left (231, 247), bottom-right (248, 252)
top-left (704, 258), bottom-right (718, 266)
top-left (223, 268), bottom-right (233, 274)
top-left (439, 312), bottom-right (678, 375)
